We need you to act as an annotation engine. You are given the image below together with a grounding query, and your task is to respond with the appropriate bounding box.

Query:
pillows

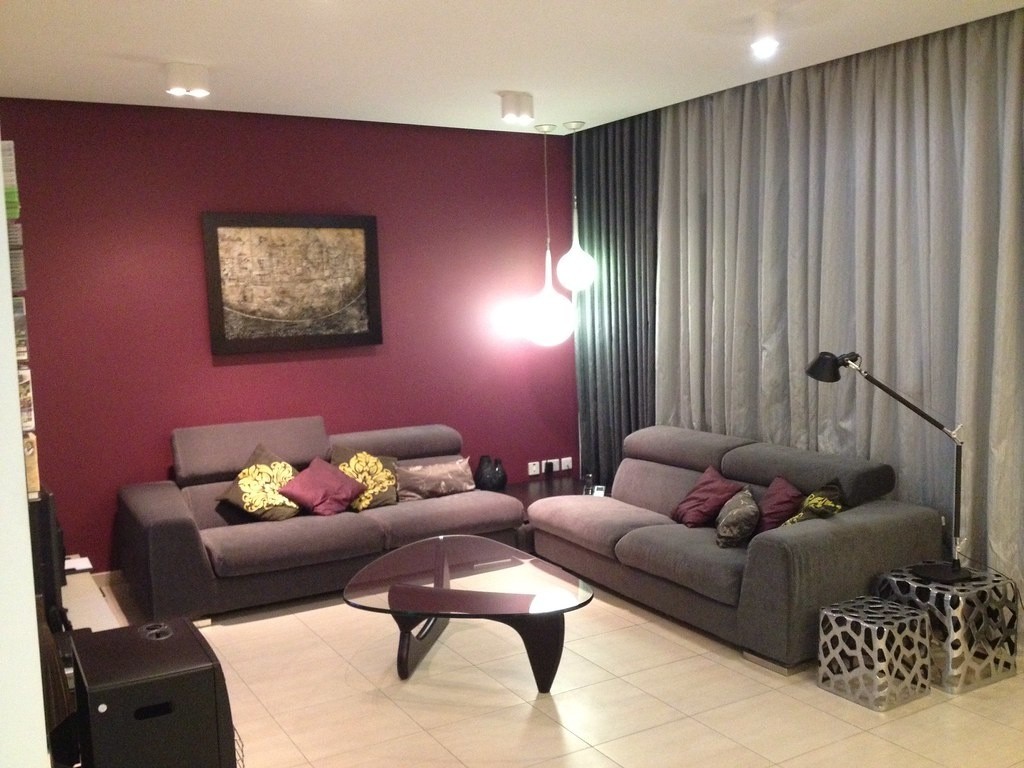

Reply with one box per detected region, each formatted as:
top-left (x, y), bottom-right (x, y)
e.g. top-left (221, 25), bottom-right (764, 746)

top-left (668, 465), bottom-right (843, 550)
top-left (215, 440), bottom-right (475, 521)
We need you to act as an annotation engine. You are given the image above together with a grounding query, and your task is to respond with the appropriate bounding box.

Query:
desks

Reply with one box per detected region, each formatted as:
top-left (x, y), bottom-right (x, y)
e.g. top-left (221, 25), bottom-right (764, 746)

top-left (342, 534), bottom-right (594, 693)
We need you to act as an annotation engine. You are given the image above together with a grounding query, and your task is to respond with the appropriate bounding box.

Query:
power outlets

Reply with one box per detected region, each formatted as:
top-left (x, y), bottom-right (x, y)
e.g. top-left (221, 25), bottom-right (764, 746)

top-left (528, 457), bottom-right (572, 476)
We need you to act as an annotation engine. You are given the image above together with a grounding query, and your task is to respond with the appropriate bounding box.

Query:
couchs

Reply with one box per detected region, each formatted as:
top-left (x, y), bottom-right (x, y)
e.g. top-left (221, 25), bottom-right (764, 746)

top-left (116, 416), bottom-right (525, 623)
top-left (527, 425), bottom-right (944, 677)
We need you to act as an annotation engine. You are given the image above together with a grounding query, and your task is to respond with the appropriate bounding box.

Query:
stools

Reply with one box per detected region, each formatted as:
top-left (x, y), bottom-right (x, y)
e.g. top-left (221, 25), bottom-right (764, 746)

top-left (817, 593), bottom-right (932, 712)
top-left (872, 558), bottom-right (1018, 694)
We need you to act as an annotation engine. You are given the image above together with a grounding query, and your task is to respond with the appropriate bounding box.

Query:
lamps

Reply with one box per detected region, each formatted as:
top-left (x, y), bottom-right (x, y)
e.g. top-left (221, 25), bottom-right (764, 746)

top-left (519, 122), bottom-right (598, 347)
top-left (750, 10), bottom-right (779, 58)
top-left (804, 351), bottom-right (860, 383)
top-left (164, 62), bottom-right (212, 98)
top-left (502, 93), bottom-right (534, 125)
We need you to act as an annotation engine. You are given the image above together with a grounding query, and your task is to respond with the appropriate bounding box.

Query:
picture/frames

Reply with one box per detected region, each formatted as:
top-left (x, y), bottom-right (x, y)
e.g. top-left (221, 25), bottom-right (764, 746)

top-left (201, 210), bottom-right (384, 356)
